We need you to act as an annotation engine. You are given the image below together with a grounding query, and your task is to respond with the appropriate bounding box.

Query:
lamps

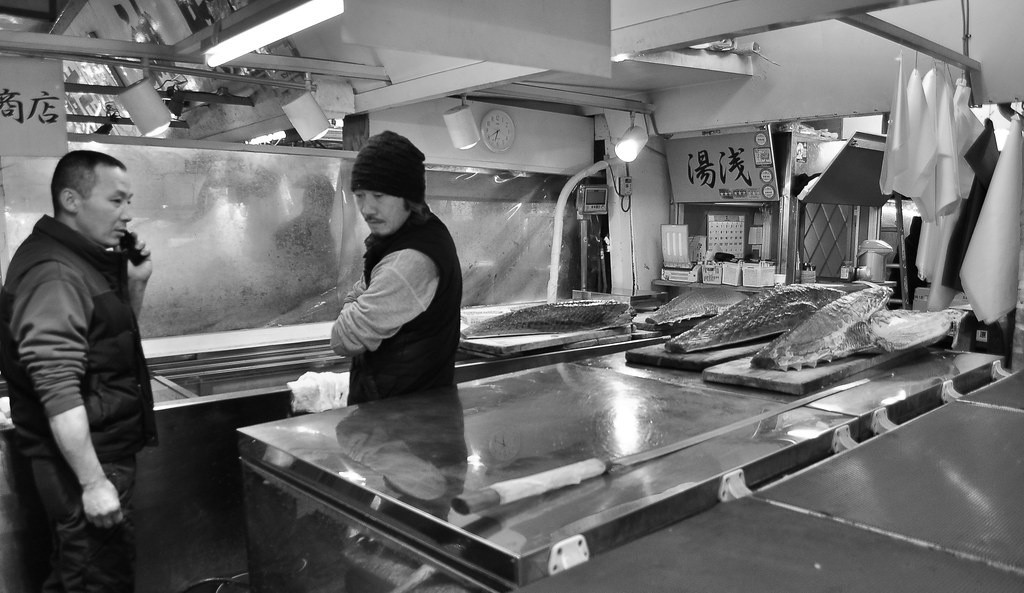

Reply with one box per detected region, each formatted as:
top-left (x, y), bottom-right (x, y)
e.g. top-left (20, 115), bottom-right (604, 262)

top-left (282, 72), bottom-right (330, 142)
top-left (114, 65), bottom-right (172, 136)
top-left (615, 111), bottom-right (648, 163)
top-left (443, 94), bottom-right (480, 150)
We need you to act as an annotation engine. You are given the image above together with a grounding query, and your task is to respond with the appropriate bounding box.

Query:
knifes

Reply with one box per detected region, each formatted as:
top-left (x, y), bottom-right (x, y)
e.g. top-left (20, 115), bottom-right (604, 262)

top-left (451, 371), bottom-right (892, 515)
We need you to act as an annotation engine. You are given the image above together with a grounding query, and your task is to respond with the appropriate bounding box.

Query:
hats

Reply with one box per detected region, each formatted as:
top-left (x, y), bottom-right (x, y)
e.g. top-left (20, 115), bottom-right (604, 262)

top-left (352, 130), bottom-right (425, 203)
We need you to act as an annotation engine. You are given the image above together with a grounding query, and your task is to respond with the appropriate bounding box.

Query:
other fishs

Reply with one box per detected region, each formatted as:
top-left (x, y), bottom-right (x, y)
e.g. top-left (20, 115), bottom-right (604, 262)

top-left (461, 284), bottom-right (957, 373)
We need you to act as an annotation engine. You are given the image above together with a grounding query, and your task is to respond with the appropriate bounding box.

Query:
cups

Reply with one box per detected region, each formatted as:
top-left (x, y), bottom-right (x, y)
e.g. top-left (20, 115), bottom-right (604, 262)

top-left (775, 274), bottom-right (786, 283)
top-left (802, 270), bottom-right (816, 283)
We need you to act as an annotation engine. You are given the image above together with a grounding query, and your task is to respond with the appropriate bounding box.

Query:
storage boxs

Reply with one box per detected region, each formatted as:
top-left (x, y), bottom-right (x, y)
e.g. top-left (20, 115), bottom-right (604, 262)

top-left (702, 264), bottom-right (775, 288)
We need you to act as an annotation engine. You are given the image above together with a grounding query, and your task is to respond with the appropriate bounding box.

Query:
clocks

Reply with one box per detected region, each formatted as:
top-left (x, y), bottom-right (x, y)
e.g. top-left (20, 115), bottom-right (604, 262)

top-left (478, 110), bottom-right (515, 153)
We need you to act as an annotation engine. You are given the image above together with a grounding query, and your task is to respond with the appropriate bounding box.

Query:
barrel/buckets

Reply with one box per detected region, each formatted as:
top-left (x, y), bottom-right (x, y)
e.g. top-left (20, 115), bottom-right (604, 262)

top-left (856, 240), bottom-right (893, 282)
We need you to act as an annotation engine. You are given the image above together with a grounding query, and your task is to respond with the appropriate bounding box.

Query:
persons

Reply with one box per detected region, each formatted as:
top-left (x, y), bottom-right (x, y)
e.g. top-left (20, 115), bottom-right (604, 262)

top-left (331, 130), bottom-right (463, 407)
top-left (0, 150), bottom-right (158, 593)
top-left (275, 181), bottom-right (335, 298)
top-left (338, 393), bottom-right (467, 521)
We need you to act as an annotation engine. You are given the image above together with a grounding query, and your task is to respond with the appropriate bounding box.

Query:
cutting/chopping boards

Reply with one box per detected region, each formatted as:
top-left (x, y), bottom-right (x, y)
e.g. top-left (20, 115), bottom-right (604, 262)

top-left (458, 326), bottom-right (637, 355)
top-left (624, 342), bottom-right (773, 372)
top-left (632, 313), bottom-right (704, 330)
top-left (702, 349), bottom-right (911, 395)
